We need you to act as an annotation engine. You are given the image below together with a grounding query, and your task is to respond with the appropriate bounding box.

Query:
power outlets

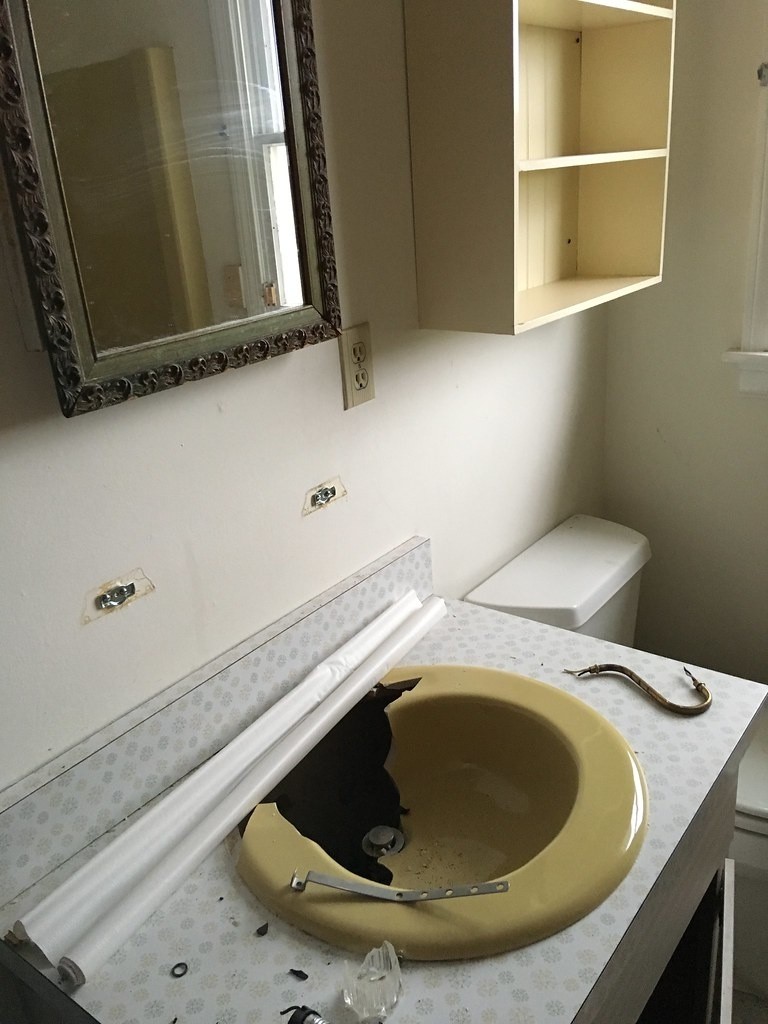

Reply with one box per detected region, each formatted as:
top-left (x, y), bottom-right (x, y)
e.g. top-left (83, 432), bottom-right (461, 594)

top-left (339, 319), bottom-right (375, 411)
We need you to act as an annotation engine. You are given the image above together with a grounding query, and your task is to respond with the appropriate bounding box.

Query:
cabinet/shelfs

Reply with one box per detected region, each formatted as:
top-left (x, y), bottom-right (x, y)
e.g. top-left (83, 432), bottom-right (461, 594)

top-left (403, 0), bottom-right (676, 336)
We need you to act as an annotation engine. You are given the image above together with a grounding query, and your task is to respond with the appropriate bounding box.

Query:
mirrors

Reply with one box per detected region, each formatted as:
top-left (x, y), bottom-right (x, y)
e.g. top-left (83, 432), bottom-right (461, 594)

top-left (0, 0), bottom-right (347, 419)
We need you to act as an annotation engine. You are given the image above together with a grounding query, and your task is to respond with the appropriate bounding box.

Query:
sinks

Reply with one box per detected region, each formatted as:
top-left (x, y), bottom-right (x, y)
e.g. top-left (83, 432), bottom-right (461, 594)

top-left (229, 664), bottom-right (648, 962)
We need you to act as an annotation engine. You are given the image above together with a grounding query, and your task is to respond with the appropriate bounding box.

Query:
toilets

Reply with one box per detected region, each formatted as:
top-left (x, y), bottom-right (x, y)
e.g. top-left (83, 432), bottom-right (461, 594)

top-left (462, 514), bottom-right (768, 1004)
